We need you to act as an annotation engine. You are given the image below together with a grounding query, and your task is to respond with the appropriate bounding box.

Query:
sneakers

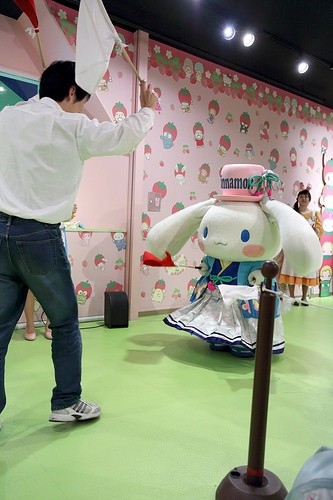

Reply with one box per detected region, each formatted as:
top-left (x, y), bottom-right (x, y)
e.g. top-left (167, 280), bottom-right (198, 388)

top-left (48, 399), bottom-right (101, 422)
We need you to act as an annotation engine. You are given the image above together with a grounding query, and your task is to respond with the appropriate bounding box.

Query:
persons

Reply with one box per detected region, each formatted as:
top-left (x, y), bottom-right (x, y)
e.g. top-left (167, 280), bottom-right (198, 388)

top-left (0, 61), bottom-right (159, 422)
top-left (24, 289), bottom-right (53, 340)
top-left (276, 190), bottom-right (323, 306)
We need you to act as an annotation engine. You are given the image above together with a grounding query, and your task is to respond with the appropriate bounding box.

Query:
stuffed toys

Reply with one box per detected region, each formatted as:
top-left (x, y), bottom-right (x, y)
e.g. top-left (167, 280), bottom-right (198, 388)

top-left (146, 164), bottom-right (324, 358)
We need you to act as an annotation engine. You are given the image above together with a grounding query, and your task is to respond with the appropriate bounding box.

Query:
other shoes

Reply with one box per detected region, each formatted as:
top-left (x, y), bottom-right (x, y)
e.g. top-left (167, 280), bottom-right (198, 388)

top-left (301, 299), bottom-right (309, 306)
top-left (294, 301), bottom-right (299, 306)
top-left (46, 331), bottom-right (53, 339)
top-left (24, 327), bottom-right (36, 341)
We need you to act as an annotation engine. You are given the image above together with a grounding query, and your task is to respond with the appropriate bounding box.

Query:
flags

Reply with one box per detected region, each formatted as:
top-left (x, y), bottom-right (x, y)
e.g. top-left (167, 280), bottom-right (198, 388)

top-left (74, 0), bottom-right (122, 95)
top-left (13, 0), bottom-right (39, 33)
